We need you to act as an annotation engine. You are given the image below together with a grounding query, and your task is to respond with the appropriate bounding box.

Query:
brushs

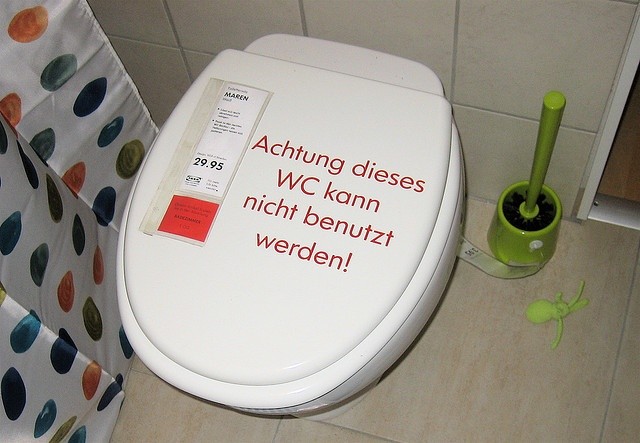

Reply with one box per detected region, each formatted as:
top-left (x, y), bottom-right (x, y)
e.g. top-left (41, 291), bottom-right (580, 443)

top-left (503, 90), bottom-right (566, 231)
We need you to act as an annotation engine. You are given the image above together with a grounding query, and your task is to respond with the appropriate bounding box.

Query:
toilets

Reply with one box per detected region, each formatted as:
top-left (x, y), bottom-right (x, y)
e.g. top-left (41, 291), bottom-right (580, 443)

top-left (114, 31), bottom-right (465, 421)
